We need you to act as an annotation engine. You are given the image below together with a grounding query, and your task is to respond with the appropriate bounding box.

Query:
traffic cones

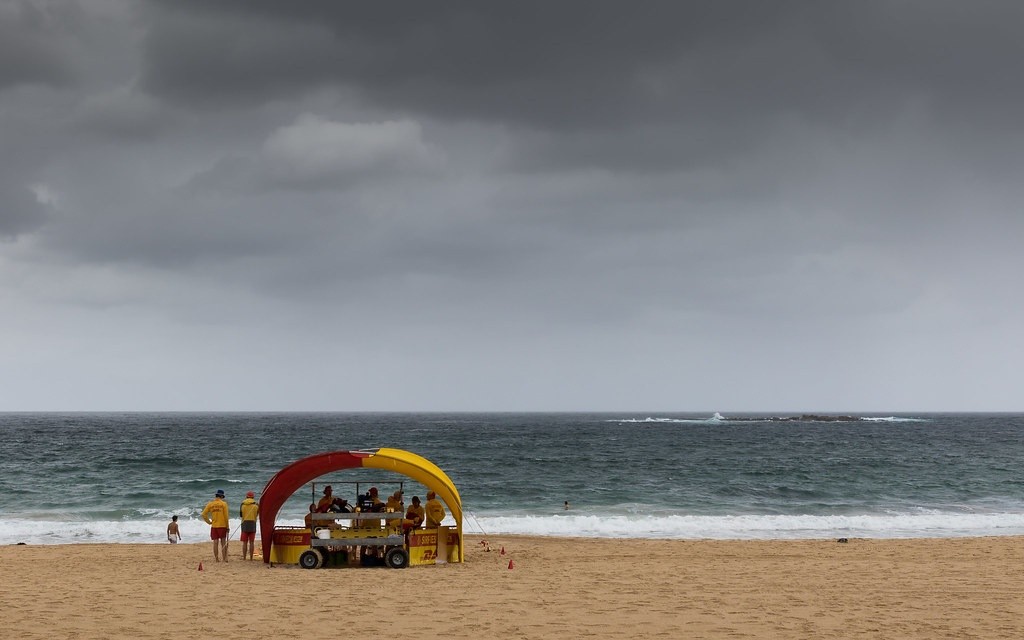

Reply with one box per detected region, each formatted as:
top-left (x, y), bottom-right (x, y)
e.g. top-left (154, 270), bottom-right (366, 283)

top-left (501, 547), bottom-right (506, 555)
top-left (198, 563), bottom-right (203, 571)
top-left (507, 558), bottom-right (514, 570)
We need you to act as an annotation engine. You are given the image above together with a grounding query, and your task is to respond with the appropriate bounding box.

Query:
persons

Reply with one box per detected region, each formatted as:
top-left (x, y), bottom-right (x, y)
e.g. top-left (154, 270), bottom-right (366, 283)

top-left (202, 490), bottom-right (230, 562)
top-left (240, 492), bottom-right (259, 562)
top-left (305, 485), bottom-right (424, 565)
top-left (564, 501), bottom-right (569, 510)
top-left (167, 516), bottom-right (181, 544)
top-left (426, 490), bottom-right (446, 528)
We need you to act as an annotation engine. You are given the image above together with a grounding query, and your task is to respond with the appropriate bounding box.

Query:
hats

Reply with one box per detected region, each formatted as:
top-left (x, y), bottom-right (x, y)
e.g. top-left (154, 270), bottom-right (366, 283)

top-left (369, 488), bottom-right (378, 498)
top-left (246, 491), bottom-right (254, 497)
top-left (412, 496), bottom-right (421, 502)
top-left (215, 490), bottom-right (225, 498)
